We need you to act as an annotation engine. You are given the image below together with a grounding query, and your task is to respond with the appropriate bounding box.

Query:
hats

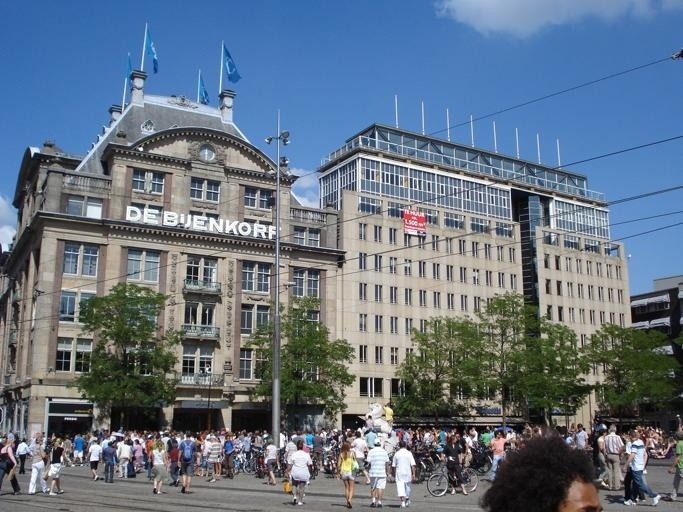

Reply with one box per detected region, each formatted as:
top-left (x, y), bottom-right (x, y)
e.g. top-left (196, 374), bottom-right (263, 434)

top-left (374, 439), bottom-right (380, 444)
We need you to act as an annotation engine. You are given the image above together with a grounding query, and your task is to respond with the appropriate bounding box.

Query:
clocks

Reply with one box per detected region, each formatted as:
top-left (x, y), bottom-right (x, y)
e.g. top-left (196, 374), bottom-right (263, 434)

top-left (198, 143), bottom-right (216, 161)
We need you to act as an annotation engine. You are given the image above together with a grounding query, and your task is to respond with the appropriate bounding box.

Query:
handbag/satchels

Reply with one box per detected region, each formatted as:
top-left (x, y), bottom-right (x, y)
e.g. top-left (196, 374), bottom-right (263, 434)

top-left (352, 461), bottom-right (359, 472)
top-left (0, 454), bottom-right (12, 474)
top-left (7, 468), bottom-right (15, 480)
top-left (284, 482), bottom-right (292, 492)
top-left (42, 464), bottom-right (50, 481)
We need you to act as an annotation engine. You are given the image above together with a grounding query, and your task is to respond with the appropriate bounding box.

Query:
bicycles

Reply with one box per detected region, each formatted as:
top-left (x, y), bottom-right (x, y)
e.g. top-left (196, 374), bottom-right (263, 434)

top-left (217, 447), bottom-right (342, 476)
top-left (388, 441), bottom-right (495, 498)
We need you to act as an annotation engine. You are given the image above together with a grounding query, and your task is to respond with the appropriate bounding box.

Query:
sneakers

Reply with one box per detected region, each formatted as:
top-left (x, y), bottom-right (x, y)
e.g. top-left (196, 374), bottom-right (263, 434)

top-left (347, 500), bottom-right (410, 508)
top-left (94, 475), bottom-right (98, 480)
top-left (43, 488), bottom-right (63, 495)
top-left (153, 480), bottom-right (193, 494)
top-left (14, 492), bottom-right (21, 495)
top-left (624, 495), bottom-right (675, 506)
top-left (293, 497), bottom-right (303, 506)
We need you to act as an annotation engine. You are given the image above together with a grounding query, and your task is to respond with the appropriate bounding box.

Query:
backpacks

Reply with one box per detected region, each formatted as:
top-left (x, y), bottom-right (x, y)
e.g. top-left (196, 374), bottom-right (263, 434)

top-left (183, 441), bottom-right (194, 461)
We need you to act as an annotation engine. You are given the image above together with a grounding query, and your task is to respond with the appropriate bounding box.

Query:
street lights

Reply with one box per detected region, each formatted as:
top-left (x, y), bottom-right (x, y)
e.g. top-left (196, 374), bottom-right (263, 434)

top-left (263, 109), bottom-right (297, 445)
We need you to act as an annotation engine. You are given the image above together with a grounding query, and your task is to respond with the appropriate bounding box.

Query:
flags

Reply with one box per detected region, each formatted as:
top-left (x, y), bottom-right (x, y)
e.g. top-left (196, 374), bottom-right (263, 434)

top-left (147, 21), bottom-right (161, 72)
top-left (200, 73), bottom-right (210, 104)
top-left (126, 53), bottom-right (135, 92)
top-left (223, 45), bottom-right (242, 82)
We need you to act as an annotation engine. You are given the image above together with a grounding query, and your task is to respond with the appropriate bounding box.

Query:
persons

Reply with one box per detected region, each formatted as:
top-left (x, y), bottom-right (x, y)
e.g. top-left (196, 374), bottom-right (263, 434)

top-left (481, 420), bottom-right (544, 465)
top-left (477, 436), bottom-right (603, 511)
top-left (544, 410), bottom-right (683, 508)
top-left (2, 402), bottom-right (480, 509)
